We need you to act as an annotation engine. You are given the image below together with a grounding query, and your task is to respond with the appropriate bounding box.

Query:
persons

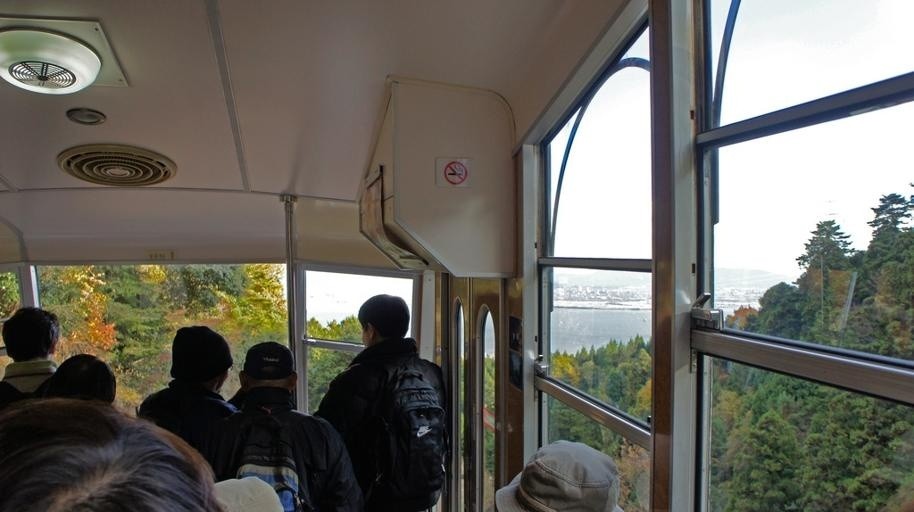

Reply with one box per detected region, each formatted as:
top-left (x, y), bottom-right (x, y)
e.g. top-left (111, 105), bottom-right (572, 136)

top-left (0, 294), bottom-right (444, 512)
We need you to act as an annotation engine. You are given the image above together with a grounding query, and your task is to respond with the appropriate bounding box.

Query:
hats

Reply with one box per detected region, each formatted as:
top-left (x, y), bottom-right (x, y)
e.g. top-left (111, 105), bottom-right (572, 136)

top-left (170, 326), bottom-right (233, 379)
top-left (245, 342), bottom-right (293, 380)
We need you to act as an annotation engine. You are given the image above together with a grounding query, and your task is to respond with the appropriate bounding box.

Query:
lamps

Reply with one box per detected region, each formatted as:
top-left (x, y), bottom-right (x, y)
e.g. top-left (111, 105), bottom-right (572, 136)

top-left (0, 16), bottom-right (132, 97)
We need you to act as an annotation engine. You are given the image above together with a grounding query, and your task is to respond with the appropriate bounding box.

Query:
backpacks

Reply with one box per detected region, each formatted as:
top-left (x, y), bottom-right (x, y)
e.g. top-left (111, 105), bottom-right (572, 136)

top-left (228, 410), bottom-right (312, 512)
top-left (356, 353), bottom-right (448, 491)
